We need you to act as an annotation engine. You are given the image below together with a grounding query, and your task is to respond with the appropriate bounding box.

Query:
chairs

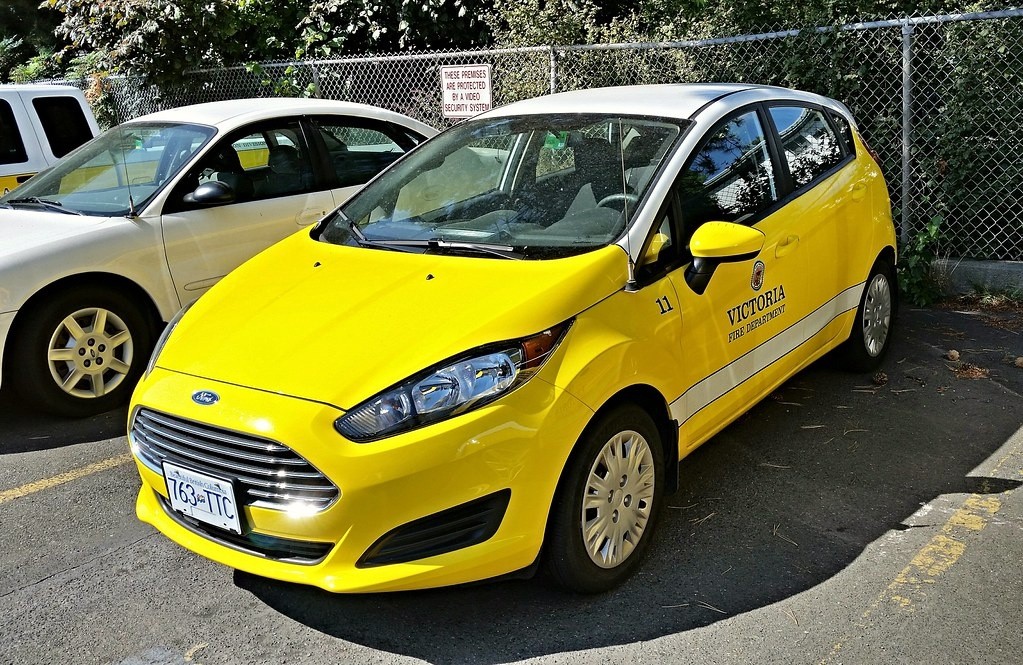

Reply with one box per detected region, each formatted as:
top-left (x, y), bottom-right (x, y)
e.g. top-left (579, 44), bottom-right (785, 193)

top-left (566, 134), bottom-right (617, 201)
top-left (190, 142), bottom-right (242, 192)
top-left (257, 146), bottom-right (301, 194)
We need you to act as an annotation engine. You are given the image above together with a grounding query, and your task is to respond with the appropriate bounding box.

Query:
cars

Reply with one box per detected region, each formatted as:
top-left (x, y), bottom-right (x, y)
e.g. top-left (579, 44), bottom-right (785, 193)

top-left (0, 98), bottom-right (510, 417)
top-left (127, 83), bottom-right (899, 594)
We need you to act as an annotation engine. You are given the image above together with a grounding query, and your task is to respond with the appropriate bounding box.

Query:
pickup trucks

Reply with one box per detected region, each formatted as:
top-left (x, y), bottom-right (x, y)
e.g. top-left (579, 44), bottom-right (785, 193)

top-left (0, 84), bottom-right (295, 198)
